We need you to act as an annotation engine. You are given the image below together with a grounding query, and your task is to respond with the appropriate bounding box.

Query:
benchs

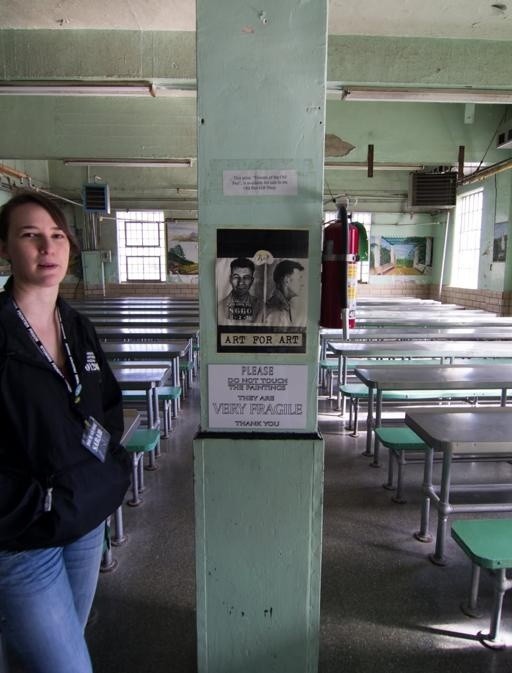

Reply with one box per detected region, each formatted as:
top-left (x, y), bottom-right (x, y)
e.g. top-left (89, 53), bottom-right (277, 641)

top-left (450, 518), bottom-right (511, 650)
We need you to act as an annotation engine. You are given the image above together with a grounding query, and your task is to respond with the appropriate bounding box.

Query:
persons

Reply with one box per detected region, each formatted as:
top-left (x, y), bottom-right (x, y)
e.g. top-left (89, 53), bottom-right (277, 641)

top-left (1, 192), bottom-right (128, 673)
top-left (264, 258), bottom-right (305, 322)
top-left (217, 258), bottom-right (261, 321)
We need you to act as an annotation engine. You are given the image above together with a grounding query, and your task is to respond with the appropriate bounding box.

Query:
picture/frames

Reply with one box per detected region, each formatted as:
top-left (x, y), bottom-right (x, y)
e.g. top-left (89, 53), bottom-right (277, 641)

top-left (165, 217), bottom-right (199, 274)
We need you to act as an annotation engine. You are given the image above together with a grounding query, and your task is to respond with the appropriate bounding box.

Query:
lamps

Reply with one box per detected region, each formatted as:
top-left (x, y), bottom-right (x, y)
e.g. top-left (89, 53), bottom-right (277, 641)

top-left (0, 84), bottom-right (155, 97)
top-left (324, 162), bottom-right (424, 170)
top-left (63, 158), bottom-right (192, 168)
top-left (340, 85), bottom-right (512, 104)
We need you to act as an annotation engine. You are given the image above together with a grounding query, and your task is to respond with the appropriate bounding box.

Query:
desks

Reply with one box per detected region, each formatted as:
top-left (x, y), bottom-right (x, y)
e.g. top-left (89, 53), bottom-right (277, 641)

top-left (62, 296), bottom-right (200, 574)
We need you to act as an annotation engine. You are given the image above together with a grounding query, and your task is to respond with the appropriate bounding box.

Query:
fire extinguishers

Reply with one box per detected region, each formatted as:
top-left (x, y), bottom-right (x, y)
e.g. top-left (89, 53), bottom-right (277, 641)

top-left (321, 197), bottom-right (359, 328)
